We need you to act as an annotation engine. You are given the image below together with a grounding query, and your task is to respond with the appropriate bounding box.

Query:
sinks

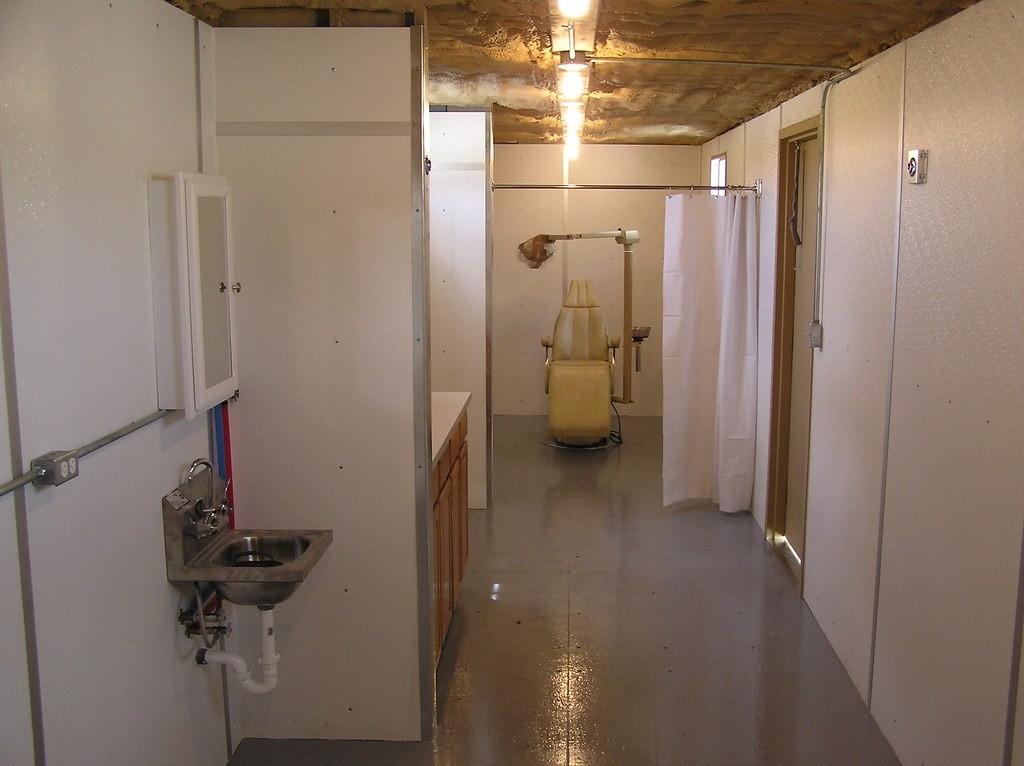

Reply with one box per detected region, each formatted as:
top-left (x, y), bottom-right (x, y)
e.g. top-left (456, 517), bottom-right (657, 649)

top-left (181, 528), bottom-right (334, 607)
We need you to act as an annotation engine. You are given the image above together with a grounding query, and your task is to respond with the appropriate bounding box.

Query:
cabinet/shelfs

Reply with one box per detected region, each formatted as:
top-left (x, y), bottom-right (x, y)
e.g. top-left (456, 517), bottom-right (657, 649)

top-left (147, 170), bottom-right (240, 419)
top-left (432, 409), bottom-right (469, 668)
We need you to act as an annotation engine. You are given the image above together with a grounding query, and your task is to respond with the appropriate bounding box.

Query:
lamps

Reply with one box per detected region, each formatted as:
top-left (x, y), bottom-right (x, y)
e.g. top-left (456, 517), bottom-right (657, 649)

top-left (558, 51), bottom-right (587, 71)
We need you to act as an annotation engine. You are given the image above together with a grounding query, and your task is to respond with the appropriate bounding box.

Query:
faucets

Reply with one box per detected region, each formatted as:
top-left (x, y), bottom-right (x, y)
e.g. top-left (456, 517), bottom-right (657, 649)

top-left (186, 457), bottom-right (216, 517)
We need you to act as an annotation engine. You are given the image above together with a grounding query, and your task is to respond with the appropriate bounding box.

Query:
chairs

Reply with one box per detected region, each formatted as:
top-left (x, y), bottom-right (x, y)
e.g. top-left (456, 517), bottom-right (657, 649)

top-left (541, 280), bottom-right (621, 450)
